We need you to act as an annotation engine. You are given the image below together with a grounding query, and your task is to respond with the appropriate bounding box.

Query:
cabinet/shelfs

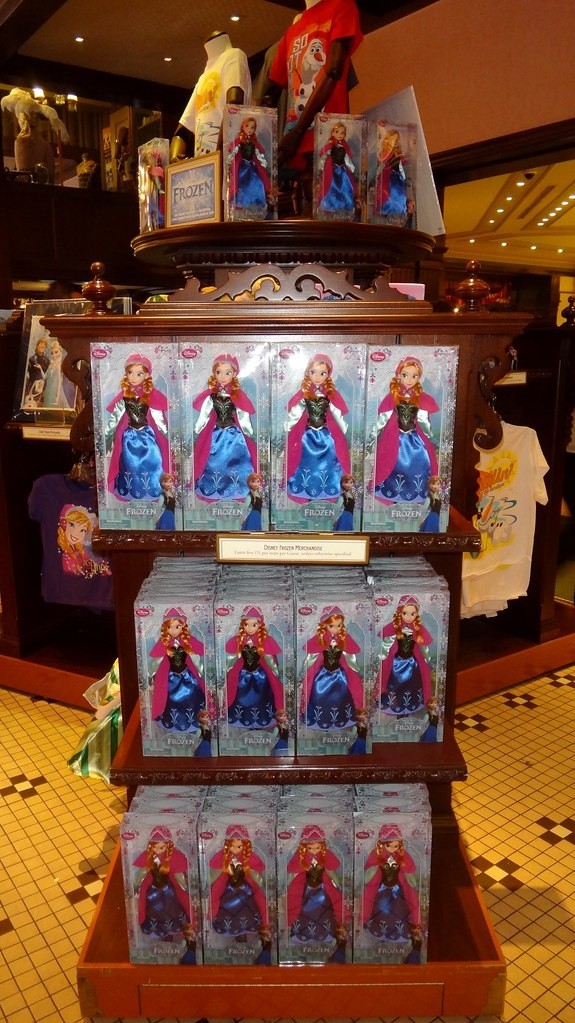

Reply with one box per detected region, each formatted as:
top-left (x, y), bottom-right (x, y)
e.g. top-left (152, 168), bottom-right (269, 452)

top-left (42, 208), bottom-right (534, 1021)
top-left (99, 105), bottom-right (163, 192)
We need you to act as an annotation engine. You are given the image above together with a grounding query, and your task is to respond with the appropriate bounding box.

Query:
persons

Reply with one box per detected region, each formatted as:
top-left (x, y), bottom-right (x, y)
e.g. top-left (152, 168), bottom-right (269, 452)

top-left (105, 354), bottom-right (441, 504)
top-left (133, 826), bottom-right (421, 965)
top-left (253, 0), bottom-right (360, 110)
top-left (319, 122), bottom-right (360, 215)
top-left (46, 280), bottom-right (83, 300)
top-left (170, 28), bottom-right (254, 201)
top-left (141, 149), bottom-right (164, 230)
top-left (228, 117), bottom-right (270, 208)
top-left (149, 595), bottom-right (434, 732)
top-left (374, 131), bottom-right (409, 218)
top-left (267, 0), bottom-right (364, 179)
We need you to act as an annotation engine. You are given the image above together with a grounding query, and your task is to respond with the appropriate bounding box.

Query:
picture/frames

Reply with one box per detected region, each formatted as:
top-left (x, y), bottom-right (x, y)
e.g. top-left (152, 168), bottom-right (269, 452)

top-left (164, 150), bottom-right (223, 227)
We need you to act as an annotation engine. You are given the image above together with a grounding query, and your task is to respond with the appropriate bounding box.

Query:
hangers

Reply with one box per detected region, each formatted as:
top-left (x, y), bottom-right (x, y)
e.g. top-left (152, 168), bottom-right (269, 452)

top-left (480, 391), bottom-right (503, 429)
top-left (70, 437), bottom-right (97, 487)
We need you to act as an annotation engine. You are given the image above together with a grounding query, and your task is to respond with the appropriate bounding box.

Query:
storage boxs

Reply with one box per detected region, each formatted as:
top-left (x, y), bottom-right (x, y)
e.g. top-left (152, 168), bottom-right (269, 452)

top-left (313, 112), bottom-right (418, 230)
top-left (120, 783), bottom-right (432, 965)
top-left (133, 557), bottom-right (450, 757)
top-left (90, 341), bottom-right (459, 534)
top-left (138, 137), bottom-right (169, 235)
top-left (222, 103), bottom-right (278, 222)
